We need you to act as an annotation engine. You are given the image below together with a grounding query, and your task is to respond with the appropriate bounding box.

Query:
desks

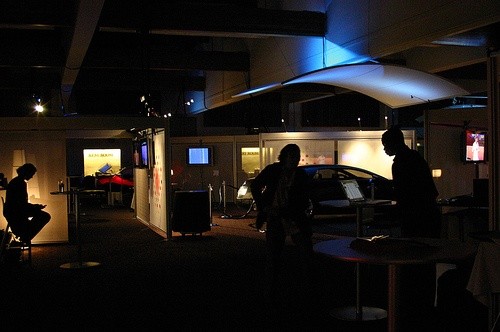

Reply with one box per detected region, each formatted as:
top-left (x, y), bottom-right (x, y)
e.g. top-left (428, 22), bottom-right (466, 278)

top-left (313, 239), bottom-right (445, 332)
top-left (442, 206), bottom-right (469, 244)
top-left (50, 190), bottom-right (105, 270)
top-left (318, 198), bottom-right (397, 237)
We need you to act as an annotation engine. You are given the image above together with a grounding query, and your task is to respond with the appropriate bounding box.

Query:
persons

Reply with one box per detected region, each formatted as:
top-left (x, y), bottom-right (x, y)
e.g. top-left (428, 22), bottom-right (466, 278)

top-left (250, 144), bottom-right (319, 291)
top-left (371, 128), bottom-right (442, 239)
top-left (3, 163), bottom-right (51, 263)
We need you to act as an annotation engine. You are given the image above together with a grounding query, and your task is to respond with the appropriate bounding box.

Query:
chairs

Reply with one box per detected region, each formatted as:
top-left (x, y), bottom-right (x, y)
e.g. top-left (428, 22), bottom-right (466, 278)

top-left (171, 190), bottom-right (211, 237)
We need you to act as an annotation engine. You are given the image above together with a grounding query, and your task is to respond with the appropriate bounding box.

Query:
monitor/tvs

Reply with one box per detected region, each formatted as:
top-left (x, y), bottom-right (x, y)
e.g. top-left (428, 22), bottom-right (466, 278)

top-left (140, 144), bottom-right (148, 167)
top-left (461, 130), bottom-right (487, 165)
top-left (187, 146), bottom-right (212, 167)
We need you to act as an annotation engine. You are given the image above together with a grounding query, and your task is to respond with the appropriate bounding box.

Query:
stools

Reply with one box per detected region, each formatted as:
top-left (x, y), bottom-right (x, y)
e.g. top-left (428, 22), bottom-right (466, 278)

top-left (1, 197), bottom-right (32, 261)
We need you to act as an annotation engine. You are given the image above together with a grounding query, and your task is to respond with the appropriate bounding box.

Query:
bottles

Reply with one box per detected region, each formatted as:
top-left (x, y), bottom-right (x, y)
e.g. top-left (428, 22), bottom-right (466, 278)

top-left (304, 198), bottom-right (313, 216)
top-left (60, 181), bottom-right (64, 192)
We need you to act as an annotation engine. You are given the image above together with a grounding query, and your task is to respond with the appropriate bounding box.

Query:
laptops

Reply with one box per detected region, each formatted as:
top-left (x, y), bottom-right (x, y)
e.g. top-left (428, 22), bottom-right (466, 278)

top-left (339, 178), bottom-right (392, 206)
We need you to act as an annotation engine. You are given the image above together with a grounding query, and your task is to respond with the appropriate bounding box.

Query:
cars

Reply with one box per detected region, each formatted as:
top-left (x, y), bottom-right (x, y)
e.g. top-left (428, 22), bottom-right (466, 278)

top-left (236, 163), bottom-right (396, 224)
